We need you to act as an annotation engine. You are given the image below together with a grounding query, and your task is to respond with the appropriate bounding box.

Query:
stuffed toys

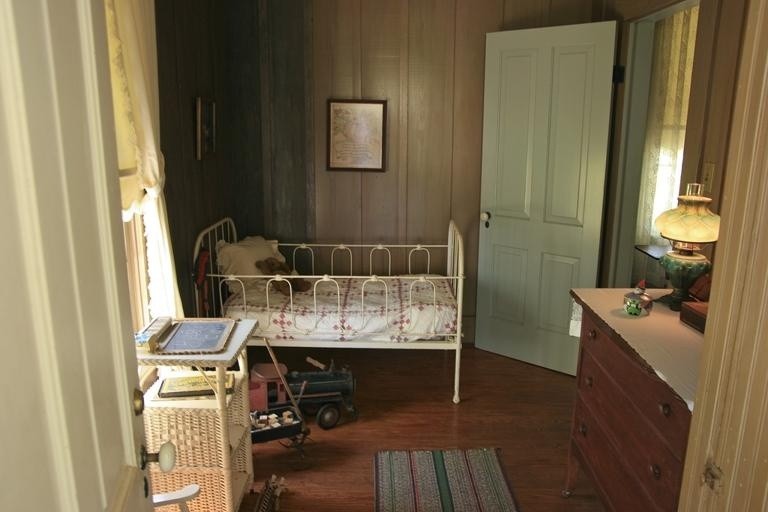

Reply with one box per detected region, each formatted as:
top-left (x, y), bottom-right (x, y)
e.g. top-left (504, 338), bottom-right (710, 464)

top-left (255, 257), bottom-right (312, 295)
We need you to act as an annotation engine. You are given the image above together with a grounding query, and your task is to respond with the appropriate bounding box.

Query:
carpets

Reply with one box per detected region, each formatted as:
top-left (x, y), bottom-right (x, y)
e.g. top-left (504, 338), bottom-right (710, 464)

top-left (372, 445), bottom-right (519, 511)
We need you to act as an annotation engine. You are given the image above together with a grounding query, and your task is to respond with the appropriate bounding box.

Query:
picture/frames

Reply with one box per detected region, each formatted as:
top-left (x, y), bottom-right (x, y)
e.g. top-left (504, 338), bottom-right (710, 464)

top-left (194, 95), bottom-right (218, 161)
top-left (326, 98), bottom-right (388, 172)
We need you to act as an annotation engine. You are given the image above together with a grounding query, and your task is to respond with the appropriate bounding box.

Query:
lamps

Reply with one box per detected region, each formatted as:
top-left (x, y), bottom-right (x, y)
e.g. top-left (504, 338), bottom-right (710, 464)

top-left (653, 182), bottom-right (722, 312)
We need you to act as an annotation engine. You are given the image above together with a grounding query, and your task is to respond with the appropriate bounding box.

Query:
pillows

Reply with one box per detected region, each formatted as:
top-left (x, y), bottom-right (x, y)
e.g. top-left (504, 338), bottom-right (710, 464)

top-left (217, 233), bottom-right (298, 302)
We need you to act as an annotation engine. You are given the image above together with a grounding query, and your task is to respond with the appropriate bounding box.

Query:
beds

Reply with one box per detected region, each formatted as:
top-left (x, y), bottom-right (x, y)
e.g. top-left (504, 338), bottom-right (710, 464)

top-left (190, 215), bottom-right (465, 405)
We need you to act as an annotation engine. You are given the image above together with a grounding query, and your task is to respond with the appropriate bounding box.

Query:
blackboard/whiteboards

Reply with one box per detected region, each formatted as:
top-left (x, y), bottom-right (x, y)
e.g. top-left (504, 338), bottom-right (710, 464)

top-left (158, 317), bottom-right (235, 354)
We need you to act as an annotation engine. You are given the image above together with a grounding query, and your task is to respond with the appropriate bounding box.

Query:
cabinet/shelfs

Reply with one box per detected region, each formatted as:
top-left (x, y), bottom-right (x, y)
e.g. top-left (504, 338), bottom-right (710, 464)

top-left (133, 315), bottom-right (253, 512)
top-left (562, 289), bottom-right (706, 511)
